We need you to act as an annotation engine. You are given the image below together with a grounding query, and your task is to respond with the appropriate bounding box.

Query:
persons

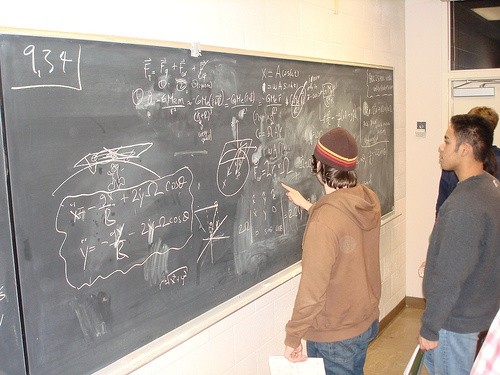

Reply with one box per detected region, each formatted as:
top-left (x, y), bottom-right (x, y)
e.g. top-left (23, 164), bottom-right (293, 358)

top-left (281, 127), bottom-right (381, 375)
top-left (435, 106), bottom-right (500, 357)
top-left (416, 113), bottom-right (500, 375)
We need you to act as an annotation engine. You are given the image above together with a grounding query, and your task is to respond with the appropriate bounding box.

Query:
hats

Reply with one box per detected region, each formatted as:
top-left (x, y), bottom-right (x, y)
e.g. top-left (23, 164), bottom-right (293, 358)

top-left (313, 126), bottom-right (358, 171)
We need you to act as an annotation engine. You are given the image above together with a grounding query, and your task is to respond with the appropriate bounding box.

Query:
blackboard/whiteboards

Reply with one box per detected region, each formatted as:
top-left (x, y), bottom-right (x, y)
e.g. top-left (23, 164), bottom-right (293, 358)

top-left (1, 27), bottom-right (397, 373)
